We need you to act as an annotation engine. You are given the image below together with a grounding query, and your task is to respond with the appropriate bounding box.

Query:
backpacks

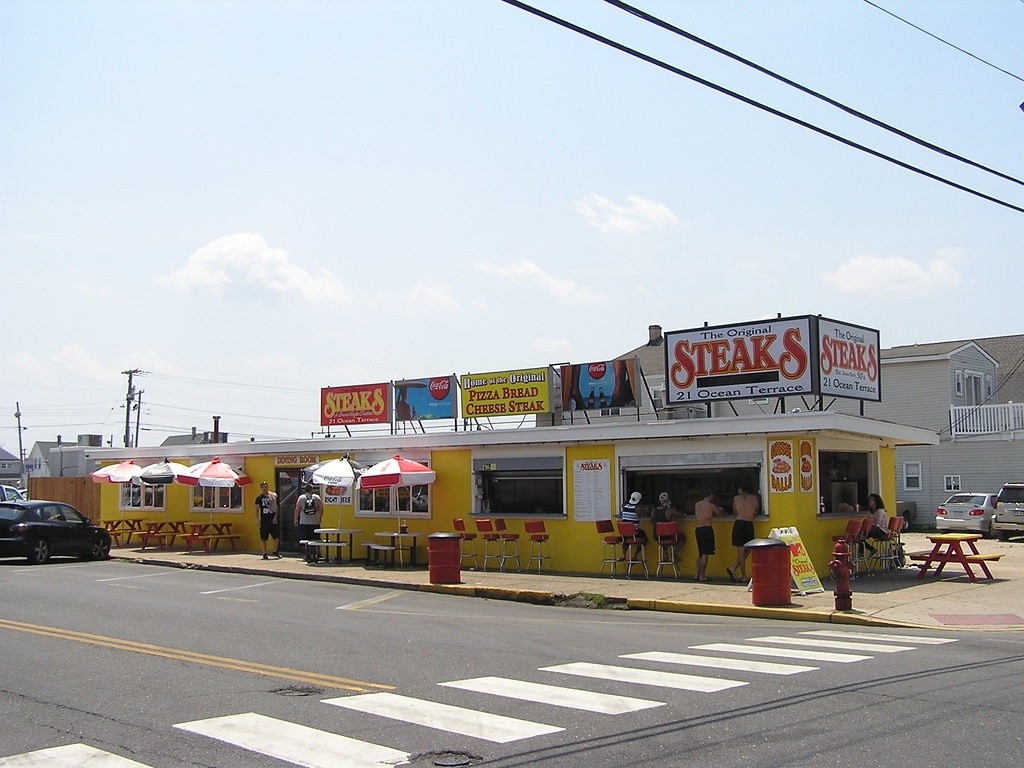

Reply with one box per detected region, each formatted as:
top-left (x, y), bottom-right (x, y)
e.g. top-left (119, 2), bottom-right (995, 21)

top-left (299, 493), bottom-right (321, 516)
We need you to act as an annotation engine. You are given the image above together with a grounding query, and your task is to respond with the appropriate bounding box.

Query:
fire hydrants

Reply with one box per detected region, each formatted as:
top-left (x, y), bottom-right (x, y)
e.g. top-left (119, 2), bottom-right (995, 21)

top-left (828, 538), bottom-right (855, 611)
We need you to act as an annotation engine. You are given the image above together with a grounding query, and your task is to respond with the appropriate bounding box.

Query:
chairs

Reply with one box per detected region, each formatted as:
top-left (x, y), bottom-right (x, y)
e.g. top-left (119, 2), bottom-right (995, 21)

top-left (597, 520), bottom-right (627, 578)
top-left (834, 515), bottom-right (906, 577)
top-left (655, 522), bottom-right (680, 582)
top-left (495, 518), bottom-right (521, 571)
top-left (618, 521), bottom-right (650, 580)
top-left (453, 518), bottom-right (478, 570)
top-left (475, 519), bottom-right (503, 571)
top-left (526, 520), bottom-right (552, 575)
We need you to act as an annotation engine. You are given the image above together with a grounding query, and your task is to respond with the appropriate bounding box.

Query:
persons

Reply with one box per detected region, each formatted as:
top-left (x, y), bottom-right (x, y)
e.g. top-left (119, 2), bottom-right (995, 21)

top-left (694, 489), bottom-right (725, 580)
top-left (255, 480), bottom-right (282, 559)
top-left (727, 485), bottom-right (758, 582)
top-left (617, 492), bottom-right (650, 561)
top-left (856, 493), bottom-right (889, 559)
top-left (652, 492), bottom-right (686, 561)
top-left (294, 484), bottom-right (324, 565)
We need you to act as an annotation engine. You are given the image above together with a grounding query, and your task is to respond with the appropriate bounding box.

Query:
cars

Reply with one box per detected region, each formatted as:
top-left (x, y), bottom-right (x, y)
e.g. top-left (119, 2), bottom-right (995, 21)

top-left (934, 492), bottom-right (999, 539)
top-left (0, 499), bottom-right (112, 564)
top-left (0, 485), bottom-right (27, 501)
top-left (896, 498), bottom-right (918, 532)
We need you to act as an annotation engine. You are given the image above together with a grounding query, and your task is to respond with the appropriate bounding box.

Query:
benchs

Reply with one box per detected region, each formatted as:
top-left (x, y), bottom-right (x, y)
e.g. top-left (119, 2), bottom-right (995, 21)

top-left (105, 528), bottom-right (245, 553)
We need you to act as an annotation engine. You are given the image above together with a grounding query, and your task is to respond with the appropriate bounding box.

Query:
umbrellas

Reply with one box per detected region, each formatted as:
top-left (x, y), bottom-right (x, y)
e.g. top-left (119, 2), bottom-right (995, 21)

top-left (176, 456), bottom-right (252, 534)
top-left (301, 458), bottom-right (370, 530)
top-left (356, 455), bottom-right (436, 566)
top-left (132, 457), bottom-right (189, 546)
top-left (88, 459), bottom-right (141, 543)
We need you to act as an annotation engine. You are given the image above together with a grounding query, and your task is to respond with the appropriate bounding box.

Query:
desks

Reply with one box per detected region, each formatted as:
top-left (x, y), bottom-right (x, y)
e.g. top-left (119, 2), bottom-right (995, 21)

top-left (103, 519), bottom-right (235, 553)
top-left (314, 527), bottom-right (362, 563)
top-left (916, 532), bottom-right (994, 584)
top-left (374, 533), bottom-right (421, 567)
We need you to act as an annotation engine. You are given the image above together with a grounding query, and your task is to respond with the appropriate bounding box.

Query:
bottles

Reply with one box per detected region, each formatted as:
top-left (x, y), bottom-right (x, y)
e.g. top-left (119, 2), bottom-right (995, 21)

top-left (587, 387), bottom-right (595, 408)
top-left (400, 525), bottom-right (408, 534)
top-left (599, 388), bottom-right (607, 408)
top-left (820, 497), bottom-right (825, 514)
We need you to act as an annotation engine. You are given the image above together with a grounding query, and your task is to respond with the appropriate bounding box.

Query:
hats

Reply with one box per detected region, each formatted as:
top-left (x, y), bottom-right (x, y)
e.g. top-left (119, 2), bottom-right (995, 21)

top-left (628, 492), bottom-right (643, 505)
top-left (658, 492), bottom-right (672, 506)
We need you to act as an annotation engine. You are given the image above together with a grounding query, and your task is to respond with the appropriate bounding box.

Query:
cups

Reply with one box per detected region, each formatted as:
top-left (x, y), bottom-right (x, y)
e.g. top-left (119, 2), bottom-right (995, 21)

top-left (855, 504), bottom-right (860, 512)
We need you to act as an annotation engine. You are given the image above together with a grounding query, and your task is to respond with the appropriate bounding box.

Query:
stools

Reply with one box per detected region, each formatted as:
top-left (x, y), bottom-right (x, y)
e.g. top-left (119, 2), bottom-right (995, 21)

top-left (361, 542), bottom-right (415, 571)
top-left (965, 554), bottom-right (1004, 583)
top-left (299, 540), bottom-right (348, 564)
top-left (904, 551), bottom-right (945, 579)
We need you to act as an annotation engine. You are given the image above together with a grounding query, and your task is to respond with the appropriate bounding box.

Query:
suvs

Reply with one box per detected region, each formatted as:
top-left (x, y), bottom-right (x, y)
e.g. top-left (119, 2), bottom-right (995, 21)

top-left (991, 482), bottom-right (1024, 543)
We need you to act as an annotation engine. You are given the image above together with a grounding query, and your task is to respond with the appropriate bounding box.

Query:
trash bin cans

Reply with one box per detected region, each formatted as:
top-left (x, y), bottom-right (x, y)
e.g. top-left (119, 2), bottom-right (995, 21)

top-left (743, 538), bottom-right (792, 605)
top-left (428, 532), bottom-right (461, 584)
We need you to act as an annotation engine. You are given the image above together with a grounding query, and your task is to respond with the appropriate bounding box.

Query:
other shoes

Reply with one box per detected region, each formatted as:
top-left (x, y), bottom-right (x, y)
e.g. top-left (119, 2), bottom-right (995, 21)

top-left (736, 575), bottom-right (750, 584)
top-left (262, 552), bottom-right (268, 560)
top-left (852, 554), bottom-right (865, 561)
top-left (725, 567), bottom-right (739, 583)
top-left (273, 550), bottom-right (283, 558)
top-left (868, 548), bottom-right (879, 561)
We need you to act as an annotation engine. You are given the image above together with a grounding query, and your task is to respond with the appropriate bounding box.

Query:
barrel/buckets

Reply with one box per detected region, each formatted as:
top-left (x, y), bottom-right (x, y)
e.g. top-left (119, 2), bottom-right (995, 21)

top-left (428, 537), bottom-right (460, 583)
top-left (751, 547), bottom-right (791, 605)
